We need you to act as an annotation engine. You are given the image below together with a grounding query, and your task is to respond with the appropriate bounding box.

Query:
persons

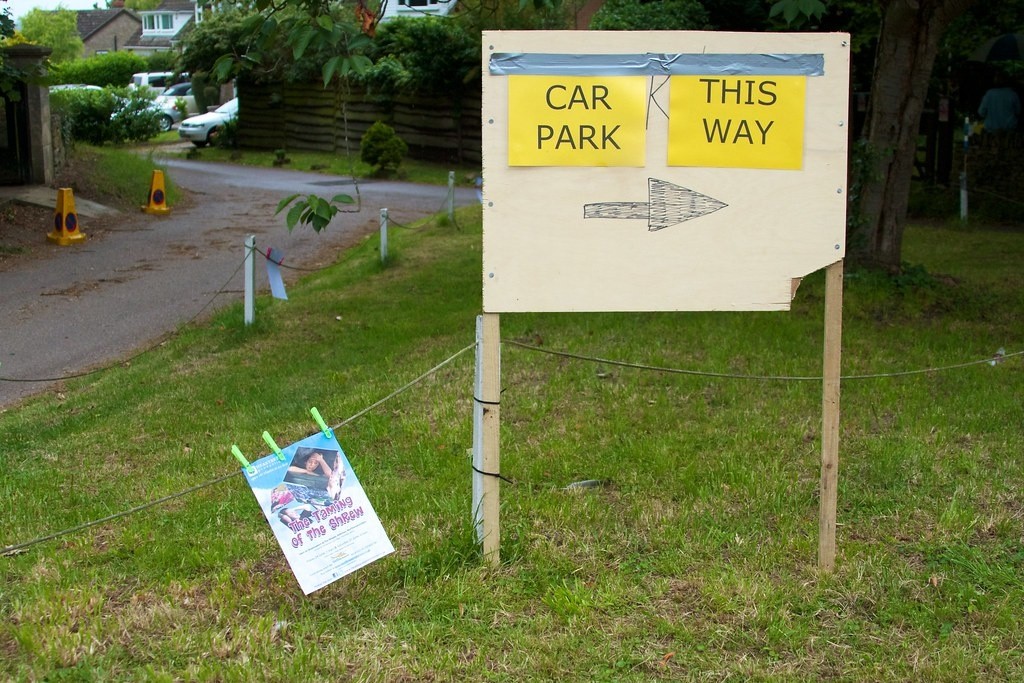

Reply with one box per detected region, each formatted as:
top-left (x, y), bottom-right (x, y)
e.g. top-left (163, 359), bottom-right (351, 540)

top-left (272, 485), bottom-right (333, 513)
top-left (287, 452), bottom-right (333, 478)
top-left (278, 504), bottom-right (313, 531)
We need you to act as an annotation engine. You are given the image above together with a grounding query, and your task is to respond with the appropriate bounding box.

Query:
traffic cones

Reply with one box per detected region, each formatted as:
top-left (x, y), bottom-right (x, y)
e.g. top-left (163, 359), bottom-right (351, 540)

top-left (141, 169), bottom-right (171, 216)
top-left (44, 189), bottom-right (87, 245)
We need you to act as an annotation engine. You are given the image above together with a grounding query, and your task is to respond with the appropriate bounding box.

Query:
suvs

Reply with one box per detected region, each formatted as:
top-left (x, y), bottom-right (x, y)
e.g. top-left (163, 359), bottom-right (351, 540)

top-left (129, 73), bottom-right (189, 96)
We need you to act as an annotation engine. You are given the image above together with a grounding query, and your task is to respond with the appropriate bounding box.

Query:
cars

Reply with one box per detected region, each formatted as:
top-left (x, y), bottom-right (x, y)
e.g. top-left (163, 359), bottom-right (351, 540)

top-left (51, 84), bottom-right (180, 132)
top-left (165, 82), bottom-right (200, 116)
top-left (175, 96), bottom-right (238, 146)
